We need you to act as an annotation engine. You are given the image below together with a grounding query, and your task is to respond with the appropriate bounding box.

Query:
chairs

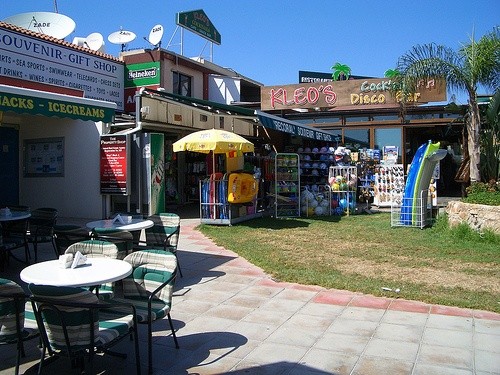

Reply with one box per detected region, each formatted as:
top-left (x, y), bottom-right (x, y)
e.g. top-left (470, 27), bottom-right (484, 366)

top-left (132, 213), bottom-right (183, 279)
top-left (51, 225), bottom-right (98, 258)
top-left (87, 228), bottom-right (135, 255)
top-left (0, 274), bottom-right (43, 375)
top-left (119, 249), bottom-right (180, 375)
top-left (63, 239), bottom-right (118, 351)
top-left (29, 206), bottom-right (59, 264)
top-left (5, 203), bottom-right (33, 263)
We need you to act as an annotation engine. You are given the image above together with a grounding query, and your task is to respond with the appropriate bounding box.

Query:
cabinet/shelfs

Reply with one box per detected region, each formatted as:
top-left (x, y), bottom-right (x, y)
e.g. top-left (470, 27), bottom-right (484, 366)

top-left (274, 153), bottom-right (302, 219)
top-left (144, 132), bottom-right (273, 227)
top-left (294, 139), bottom-right (447, 230)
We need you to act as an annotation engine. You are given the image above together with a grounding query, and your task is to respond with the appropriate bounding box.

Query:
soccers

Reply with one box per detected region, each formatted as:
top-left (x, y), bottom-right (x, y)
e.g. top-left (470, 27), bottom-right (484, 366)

top-left (301, 173), bottom-right (356, 215)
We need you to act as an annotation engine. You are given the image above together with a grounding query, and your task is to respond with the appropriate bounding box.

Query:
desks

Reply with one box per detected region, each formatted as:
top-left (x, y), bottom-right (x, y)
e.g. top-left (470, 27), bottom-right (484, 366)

top-left (19, 251), bottom-right (134, 375)
top-left (87, 219), bottom-right (154, 250)
top-left (0, 207), bottom-right (32, 264)
